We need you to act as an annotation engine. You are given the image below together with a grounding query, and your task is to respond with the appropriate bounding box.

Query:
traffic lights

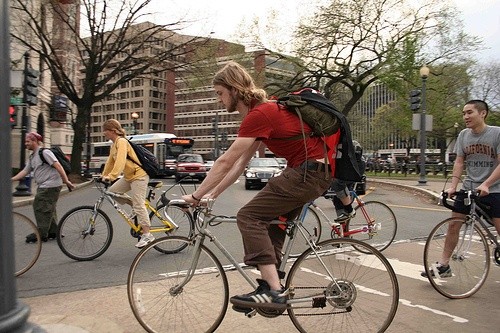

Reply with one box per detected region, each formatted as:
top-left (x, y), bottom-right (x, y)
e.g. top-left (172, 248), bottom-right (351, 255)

top-left (8, 105), bottom-right (19, 127)
top-left (408, 90), bottom-right (422, 111)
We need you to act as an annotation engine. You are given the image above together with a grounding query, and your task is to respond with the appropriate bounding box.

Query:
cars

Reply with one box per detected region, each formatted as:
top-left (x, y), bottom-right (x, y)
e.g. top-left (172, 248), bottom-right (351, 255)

top-left (204, 160), bottom-right (214, 169)
top-left (241, 157), bottom-right (287, 190)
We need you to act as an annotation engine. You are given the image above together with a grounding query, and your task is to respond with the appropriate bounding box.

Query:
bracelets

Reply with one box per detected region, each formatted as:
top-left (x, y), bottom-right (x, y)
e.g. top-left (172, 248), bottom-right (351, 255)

top-left (192, 193), bottom-right (200, 203)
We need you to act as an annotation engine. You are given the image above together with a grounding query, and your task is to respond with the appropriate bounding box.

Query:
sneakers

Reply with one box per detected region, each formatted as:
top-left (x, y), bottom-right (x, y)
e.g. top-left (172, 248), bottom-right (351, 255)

top-left (129, 209), bottom-right (137, 219)
top-left (135, 232), bottom-right (155, 247)
top-left (334, 208), bottom-right (356, 222)
top-left (230, 279), bottom-right (291, 308)
top-left (421, 262), bottom-right (452, 278)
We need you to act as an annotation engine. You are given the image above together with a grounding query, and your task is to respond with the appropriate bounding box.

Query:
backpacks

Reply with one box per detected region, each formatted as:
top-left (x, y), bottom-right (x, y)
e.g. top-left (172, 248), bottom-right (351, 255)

top-left (38, 147), bottom-right (72, 176)
top-left (266, 88), bottom-right (362, 182)
top-left (116, 136), bottom-right (160, 175)
top-left (352, 140), bottom-right (365, 175)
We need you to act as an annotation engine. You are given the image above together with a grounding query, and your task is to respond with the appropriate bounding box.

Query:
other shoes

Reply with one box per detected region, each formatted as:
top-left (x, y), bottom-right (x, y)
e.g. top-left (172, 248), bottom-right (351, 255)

top-left (48, 232), bottom-right (57, 239)
top-left (26, 233), bottom-right (48, 243)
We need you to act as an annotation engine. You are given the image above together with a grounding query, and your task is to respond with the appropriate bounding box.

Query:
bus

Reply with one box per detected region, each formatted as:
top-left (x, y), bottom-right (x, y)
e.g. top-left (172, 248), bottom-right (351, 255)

top-left (88, 133), bottom-right (194, 178)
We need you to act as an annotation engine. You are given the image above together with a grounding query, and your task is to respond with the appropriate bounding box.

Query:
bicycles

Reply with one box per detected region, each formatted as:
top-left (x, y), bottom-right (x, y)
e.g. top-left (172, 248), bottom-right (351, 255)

top-left (54, 176), bottom-right (194, 262)
top-left (282, 181), bottom-right (397, 257)
top-left (422, 189), bottom-right (498, 301)
top-left (126, 174), bottom-right (400, 333)
top-left (13, 212), bottom-right (43, 278)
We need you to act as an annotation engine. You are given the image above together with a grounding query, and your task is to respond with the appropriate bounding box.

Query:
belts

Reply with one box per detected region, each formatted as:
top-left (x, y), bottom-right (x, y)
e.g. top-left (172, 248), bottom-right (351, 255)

top-left (301, 163), bottom-right (331, 172)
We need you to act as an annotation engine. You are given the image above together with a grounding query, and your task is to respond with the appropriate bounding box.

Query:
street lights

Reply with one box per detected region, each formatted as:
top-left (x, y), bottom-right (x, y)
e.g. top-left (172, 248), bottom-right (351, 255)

top-left (415, 58), bottom-right (431, 186)
top-left (131, 112), bottom-right (139, 134)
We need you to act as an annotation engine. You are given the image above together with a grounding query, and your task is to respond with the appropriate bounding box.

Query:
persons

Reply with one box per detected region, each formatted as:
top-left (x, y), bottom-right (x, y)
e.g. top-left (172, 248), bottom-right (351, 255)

top-left (99, 119), bottom-right (155, 248)
top-left (421, 100), bottom-right (500, 279)
top-left (180, 61), bottom-right (333, 309)
top-left (11, 132), bottom-right (77, 243)
top-left (332, 157), bottom-right (366, 246)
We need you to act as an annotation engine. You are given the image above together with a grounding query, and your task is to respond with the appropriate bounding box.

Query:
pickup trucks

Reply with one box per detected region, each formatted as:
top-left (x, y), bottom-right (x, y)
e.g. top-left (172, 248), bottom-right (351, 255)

top-left (173, 153), bottom-right (206, 182)
